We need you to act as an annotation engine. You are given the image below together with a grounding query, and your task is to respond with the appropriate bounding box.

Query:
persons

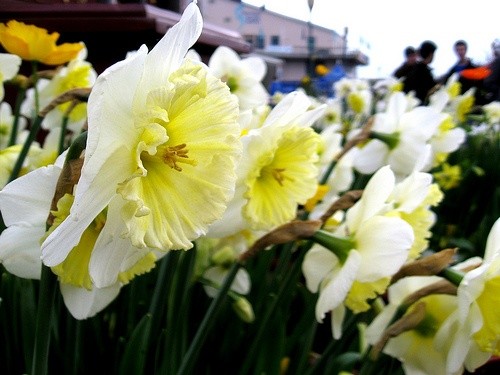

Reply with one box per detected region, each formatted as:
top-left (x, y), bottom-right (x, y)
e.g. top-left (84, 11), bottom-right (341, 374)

top-left (409, 40), bottom-right (442, 107)
top-left (480, 39), bottom-right (500, 102)
top-left (390, 46), bottom-right (418, 96)
top-left (441, 39), bottom-right (486, 107)
